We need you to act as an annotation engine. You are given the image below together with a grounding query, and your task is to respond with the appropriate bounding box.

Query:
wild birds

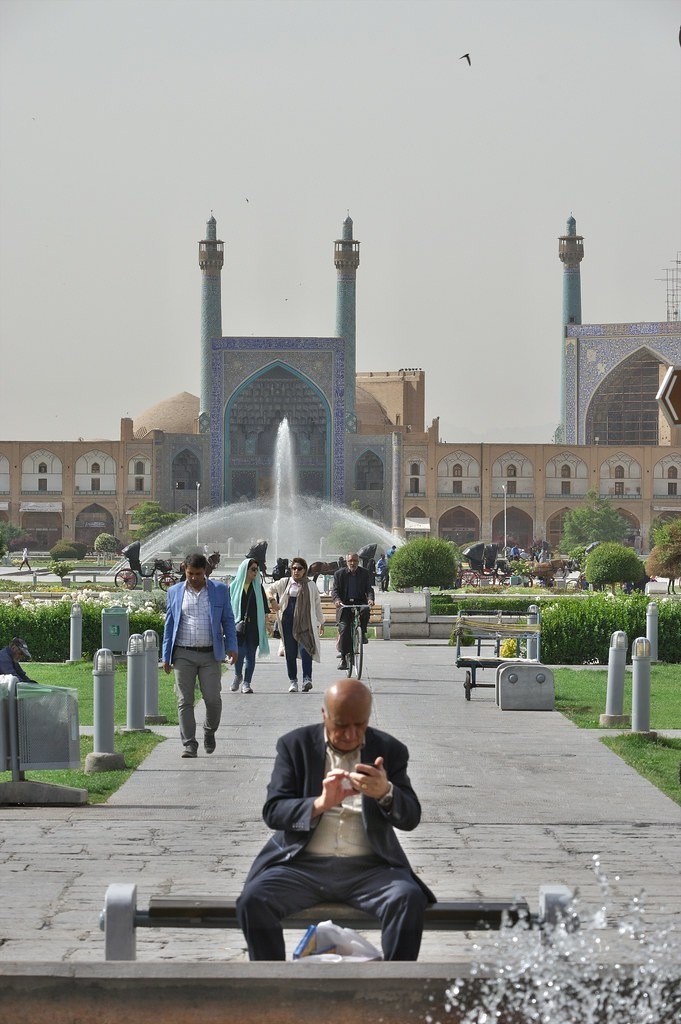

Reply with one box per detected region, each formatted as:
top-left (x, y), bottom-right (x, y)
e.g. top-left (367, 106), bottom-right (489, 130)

top-left (459, 53), bottom-right (471, 66)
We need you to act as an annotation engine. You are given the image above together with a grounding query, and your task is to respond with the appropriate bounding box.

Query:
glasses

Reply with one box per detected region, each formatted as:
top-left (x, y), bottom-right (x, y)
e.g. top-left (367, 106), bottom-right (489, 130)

top-left (292, 567), bottom-right (304, 571)
top-left (248, 567), bottom-right (258, 571)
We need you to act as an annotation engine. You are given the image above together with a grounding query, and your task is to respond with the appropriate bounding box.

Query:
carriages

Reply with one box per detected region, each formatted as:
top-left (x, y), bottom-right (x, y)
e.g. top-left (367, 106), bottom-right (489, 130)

top-left (113, 540), bottom-right (221, 593)
top-left (459, 543), bottom-right (573, 590)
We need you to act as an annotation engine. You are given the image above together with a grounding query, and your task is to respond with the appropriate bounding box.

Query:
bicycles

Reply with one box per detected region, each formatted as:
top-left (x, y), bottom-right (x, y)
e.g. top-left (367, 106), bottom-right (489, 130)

top-left (339, 602), bottom-right (374, 680)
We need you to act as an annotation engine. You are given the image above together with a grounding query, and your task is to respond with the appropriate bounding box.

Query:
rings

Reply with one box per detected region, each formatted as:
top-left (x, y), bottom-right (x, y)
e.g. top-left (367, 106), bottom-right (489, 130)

top-left (361, 783), bottom-right (368, 790)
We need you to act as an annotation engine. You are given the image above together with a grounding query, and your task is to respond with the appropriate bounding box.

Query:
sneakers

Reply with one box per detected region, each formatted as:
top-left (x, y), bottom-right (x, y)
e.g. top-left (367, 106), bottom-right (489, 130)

top-left (241, 683), bottom-right (253, 693)
top-left (182, 741), bottom-right (198, 758)
top-left (204, 730), bottom-right (216, 753)
top-left (302, 681), bottom-right (312, 692)
top-left (231, 675), bottom-right (243, 691)
top-left (289, 679), bottom-right (298, 692)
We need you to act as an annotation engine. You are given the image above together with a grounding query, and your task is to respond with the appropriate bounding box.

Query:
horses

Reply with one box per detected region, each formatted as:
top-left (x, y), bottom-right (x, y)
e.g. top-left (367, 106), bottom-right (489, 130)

top-left (520, 558), bottom-right (570, 587)
top-left (180, 551), bottom-right (220, 580)
top-left (307, 561), bottom-right (346, 584)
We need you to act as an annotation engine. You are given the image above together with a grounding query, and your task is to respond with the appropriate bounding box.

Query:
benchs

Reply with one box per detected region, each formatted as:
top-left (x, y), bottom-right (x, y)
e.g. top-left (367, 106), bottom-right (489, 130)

top-left (98, 882), bottom-right (579, 963)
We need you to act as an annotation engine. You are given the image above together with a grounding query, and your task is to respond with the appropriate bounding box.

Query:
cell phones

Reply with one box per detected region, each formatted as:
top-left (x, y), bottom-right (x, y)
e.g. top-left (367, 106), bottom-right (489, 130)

top-left (353, 764), bottom-right (378, 792)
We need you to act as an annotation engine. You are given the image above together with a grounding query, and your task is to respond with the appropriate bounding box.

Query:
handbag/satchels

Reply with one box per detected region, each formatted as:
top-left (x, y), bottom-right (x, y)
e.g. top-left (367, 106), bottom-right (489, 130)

top-left (297, 920), bottom-right (382, 964)
top-left (273, 620), bottom-right (281, 638)
top-left (235, 620), bottom-right (245, 646)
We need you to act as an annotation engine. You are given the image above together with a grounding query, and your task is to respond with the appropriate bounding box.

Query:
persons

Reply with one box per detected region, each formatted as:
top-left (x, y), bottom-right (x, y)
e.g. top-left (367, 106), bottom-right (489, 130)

top-left (368, 557), bottom-right (376, 586)
top-left (228, 558), bottom-right (273, 695)
top-left (377, 554), bottom-right (390, 591)
top-left (650, 575), bottom-right (657, 582)
top-left (0, 637), bottom-right (38, 683)
top-left (204, 544), bottom-right (208, 552)
top-left (17, 548), bottom-right (31, 570)
top-left (337, 557), bottom-right (346, 568)
top-left (622, 582), bottom-right (628, 594)
top-left (333, 553), bottom-right (374, 672)
top-left (581, 576), bottom-right (589, 589)
top-left (387, 546), bottom-right (396, 557)
top-left (267, 558), bottom-right (327, 693)
top-left (510, 544), bottom-right (520, 561)
top-left (529, 536), bottom-right (550, 562)
top-left (235, 678), bottom-right (437, 961)
top-left (161, 554), bottom-right (236, 757)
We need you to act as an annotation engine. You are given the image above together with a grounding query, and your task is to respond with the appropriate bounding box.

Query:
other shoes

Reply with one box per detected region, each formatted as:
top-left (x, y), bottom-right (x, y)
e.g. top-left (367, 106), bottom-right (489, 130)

top-left (337, 659), bottom-right (347, 669)
top-left (362, 635), bottom-right (368, 644)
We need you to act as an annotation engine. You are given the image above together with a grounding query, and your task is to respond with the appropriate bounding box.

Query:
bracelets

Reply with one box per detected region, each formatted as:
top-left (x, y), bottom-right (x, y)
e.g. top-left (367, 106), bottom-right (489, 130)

top-left (270, 598), bottom-right (275, 603)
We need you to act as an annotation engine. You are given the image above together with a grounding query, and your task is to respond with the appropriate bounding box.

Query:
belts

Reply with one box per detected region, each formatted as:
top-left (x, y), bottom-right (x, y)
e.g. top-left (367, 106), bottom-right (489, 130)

top-left (345, 598), bottom-right (363, 603)
top-left (176, 645), bottom-right (213, 652)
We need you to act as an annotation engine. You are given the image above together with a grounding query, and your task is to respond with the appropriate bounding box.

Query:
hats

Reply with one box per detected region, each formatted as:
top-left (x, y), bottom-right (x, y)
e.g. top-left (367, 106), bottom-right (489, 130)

top-left (13, 637), bottom-right (31, 658)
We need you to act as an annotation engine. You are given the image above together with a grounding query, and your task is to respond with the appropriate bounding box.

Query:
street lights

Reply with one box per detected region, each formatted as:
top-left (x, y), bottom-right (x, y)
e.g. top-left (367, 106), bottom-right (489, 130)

top-left (501, 485), bottom-right (508, 560)
top-left (195, 482), bottom-right (201, 546)
